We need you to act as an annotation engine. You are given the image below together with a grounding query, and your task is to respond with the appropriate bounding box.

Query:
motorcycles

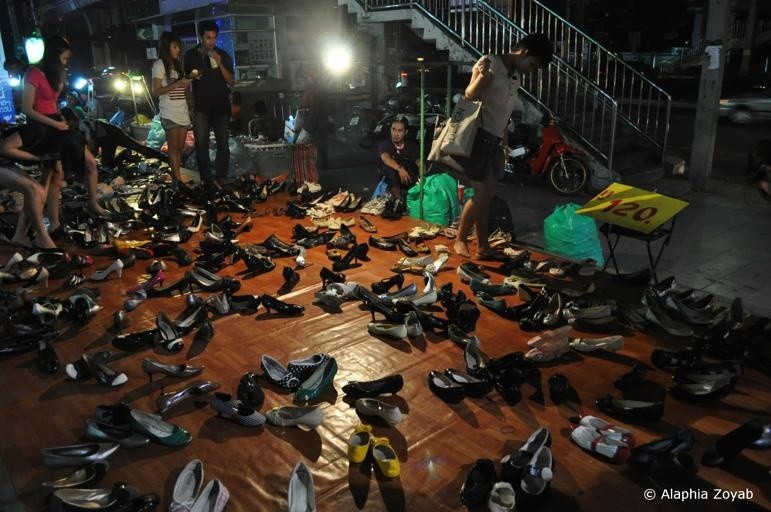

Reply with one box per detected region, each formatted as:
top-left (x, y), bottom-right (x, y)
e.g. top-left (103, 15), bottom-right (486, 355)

top-left (501, 111), bottom-right (591, 196)
top-left (337, 90), bottom-right (465, 153)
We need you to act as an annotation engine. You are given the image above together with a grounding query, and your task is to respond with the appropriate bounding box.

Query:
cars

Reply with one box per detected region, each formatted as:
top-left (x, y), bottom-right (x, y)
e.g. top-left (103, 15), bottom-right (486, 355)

top-left (100, 66), bottom-right (121, 79)
top-left (719, 84), bottom-right (771, 128)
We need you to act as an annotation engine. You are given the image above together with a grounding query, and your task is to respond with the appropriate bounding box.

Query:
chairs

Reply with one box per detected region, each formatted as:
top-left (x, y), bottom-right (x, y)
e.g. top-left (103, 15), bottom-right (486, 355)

top-left (597, 192), bottom-right (681, 284)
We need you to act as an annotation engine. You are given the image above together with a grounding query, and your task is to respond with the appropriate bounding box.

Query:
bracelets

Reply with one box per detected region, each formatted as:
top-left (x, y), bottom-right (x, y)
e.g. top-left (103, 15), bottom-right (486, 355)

top-left (396, 165), bottom-right (403, 175)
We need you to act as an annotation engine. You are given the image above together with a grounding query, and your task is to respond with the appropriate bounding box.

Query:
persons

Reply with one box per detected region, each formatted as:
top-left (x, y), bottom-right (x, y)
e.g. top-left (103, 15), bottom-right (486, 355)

top-left (0, 107), bottom-right (80, 248)
top-left (452, 31), bottom-right (554, 259)
top-left (151, 31), bottom-right (192, 183)
top-left (2, 54), bottom-right (196, 156)
top-left (230, 68), bottom-right (351, 170)
top-left (61, 107), bottom-right (169, 168)
top-left (20, 34), bottom-right (113, 233)
top-left (376, 115), bottom-right (426, 216)
top-left (182, 19), bottom-right (235, 188)
top-left (745, 137), bottom-right (770, 203)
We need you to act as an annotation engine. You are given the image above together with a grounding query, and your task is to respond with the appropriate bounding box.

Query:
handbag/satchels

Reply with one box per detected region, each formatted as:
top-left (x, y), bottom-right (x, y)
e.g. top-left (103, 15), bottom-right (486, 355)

top-left (426, 97), bottom-right (502, 181)
